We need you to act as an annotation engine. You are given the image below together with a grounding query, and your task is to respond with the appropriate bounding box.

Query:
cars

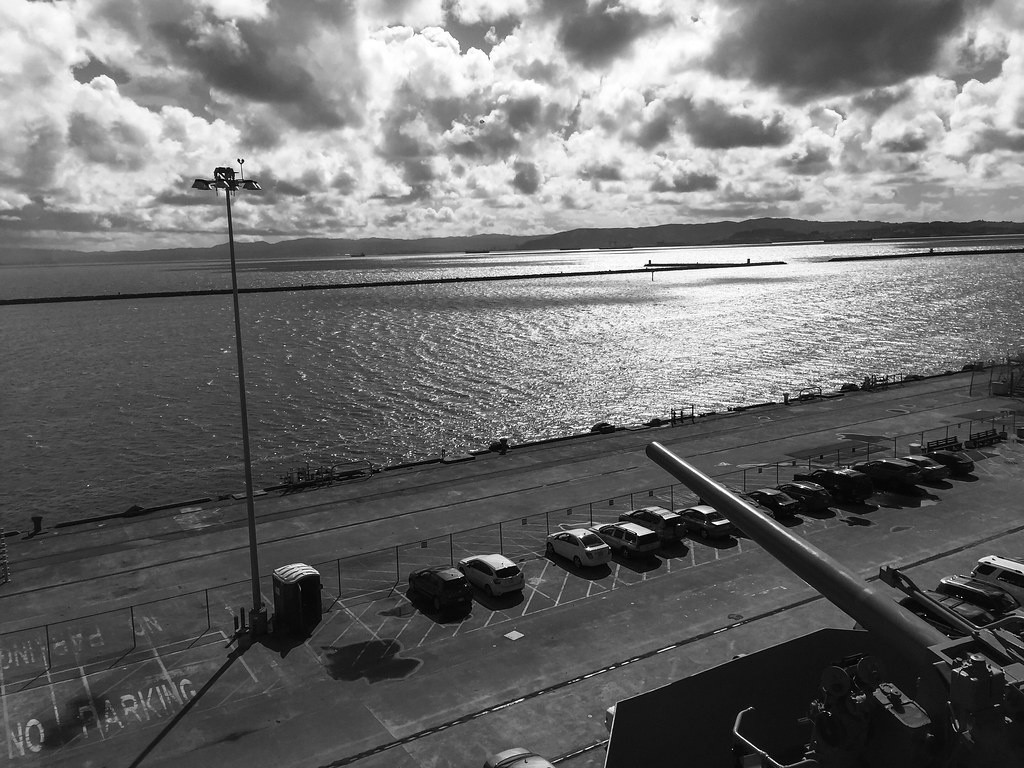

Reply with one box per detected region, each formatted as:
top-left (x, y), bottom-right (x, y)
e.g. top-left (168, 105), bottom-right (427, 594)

top-left (730, 488), bottom-right (776, 522)
top-left (457, 553), bottom-right (526, 599)
top-left (854, 555), bottom-right (1024, 740)
top-left (409, 564), bottom-right (473, 612)
top-left (744, 488), bottom-right (801, 522)
top-left (672, 504), bottom-right (736, 541)
top-left (900, 455), bottom-right (951, 484)
top-left (483, 747), bottom-right (557, 768)
top-left (926, 449), bottom-right (975, 478)
top-left (546, 528), bottom-right (613, 569)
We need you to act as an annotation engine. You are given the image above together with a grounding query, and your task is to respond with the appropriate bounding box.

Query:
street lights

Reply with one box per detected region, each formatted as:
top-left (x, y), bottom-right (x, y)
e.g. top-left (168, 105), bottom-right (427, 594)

top-left (191, 159), bottom-right (268, 639)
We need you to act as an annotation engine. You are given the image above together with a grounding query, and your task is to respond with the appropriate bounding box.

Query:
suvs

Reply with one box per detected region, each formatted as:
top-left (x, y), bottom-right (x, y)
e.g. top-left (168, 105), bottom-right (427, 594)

top-left (849, 458), bottom-right (924, 491)
top-left (588, 520), bottom-right (662, 559)
top-left (775, 480), bottom-right (833, 515)
top-left (618, 506), bottom-right (688, 549)
top-left (794, 466), bottom-right (874, 504)
